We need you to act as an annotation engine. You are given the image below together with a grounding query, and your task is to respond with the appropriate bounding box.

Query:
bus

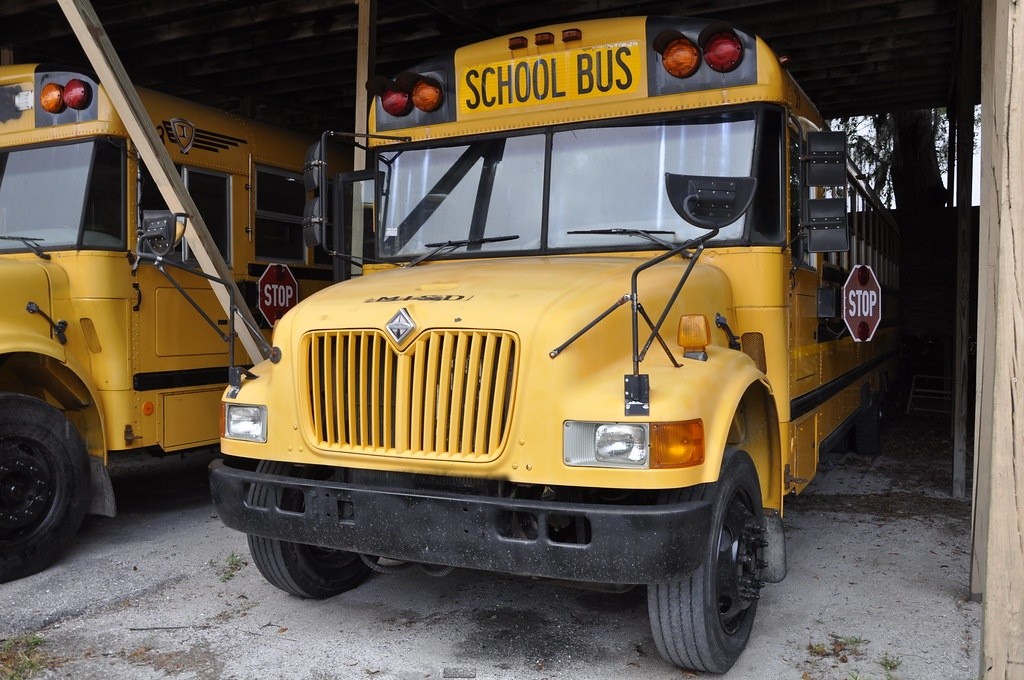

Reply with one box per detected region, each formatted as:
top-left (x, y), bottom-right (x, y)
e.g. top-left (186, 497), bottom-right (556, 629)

top-left (0, 62), bottom-right (361, 583)
top-left (209, 14), bottom-right (902, 673)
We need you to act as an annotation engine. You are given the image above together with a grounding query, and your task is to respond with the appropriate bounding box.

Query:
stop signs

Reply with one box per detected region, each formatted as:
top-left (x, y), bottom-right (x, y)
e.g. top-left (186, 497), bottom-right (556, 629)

top-left (257, 264), bottom-right (299, 330)
top-left (842, 265), bottom-right (883, 342)
